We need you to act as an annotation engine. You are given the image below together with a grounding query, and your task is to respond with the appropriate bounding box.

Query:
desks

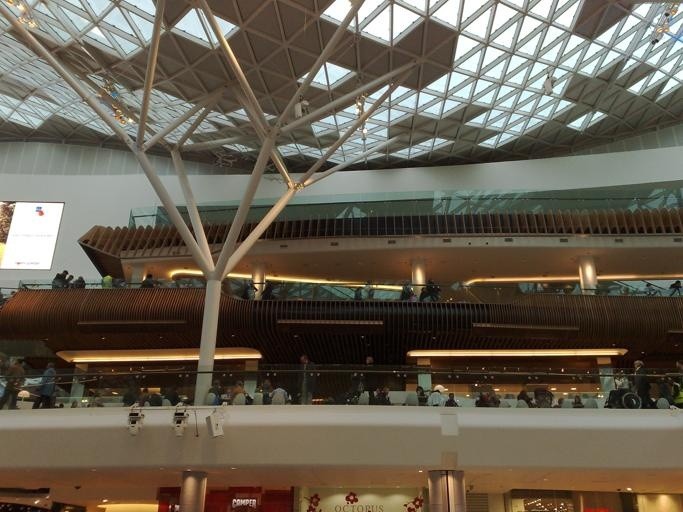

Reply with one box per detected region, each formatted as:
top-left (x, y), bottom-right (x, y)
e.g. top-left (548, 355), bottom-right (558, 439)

top-left (358, 390), bottom-right (446, 406)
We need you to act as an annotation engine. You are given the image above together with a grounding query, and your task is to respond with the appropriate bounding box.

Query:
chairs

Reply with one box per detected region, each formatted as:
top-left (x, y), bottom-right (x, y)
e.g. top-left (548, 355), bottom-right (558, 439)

top-left (144, 392), bottom-right (284, 406)
top-left (405, 391), bottom-right (598, 408)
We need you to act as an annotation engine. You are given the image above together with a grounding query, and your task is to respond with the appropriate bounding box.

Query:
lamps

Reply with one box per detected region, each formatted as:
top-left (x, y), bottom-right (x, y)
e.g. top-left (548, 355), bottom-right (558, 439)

top-left (292, 98), bottom-right (311, 119)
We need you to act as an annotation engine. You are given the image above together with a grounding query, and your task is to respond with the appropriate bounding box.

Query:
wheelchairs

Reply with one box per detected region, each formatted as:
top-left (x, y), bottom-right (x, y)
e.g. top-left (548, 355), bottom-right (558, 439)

top-left (604, 384), bottom-right (643, 409)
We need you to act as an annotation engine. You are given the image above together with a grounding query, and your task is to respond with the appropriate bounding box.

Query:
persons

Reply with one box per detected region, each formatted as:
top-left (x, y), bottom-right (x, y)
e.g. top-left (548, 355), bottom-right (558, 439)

top-left (51, 270), bottom-right (86, 289)
top-left (33, 362), bottom-right (56, 408)
top-left (352, 356), bottom-right (383, 381)
top-left (400, 278), bottom-right (442, 301)
top-left (102, 271), bottom-right (114, 288)
top-left (474, 388), bottom-right (587, 408)
top-left (0, 356), bottom-right (25, 410)
top-left (206, 382), bottom-right (289, 405)
top-left (139, 274), bottom-right (161, 288)
top-left (300, 355), bottom-right (317, 405)
top-left (369, 385), bottom-right (459, 406)
top-left (122, 382), bottom-right (181, 406)
top-left (604, 360), bottom-right (683, 409)
